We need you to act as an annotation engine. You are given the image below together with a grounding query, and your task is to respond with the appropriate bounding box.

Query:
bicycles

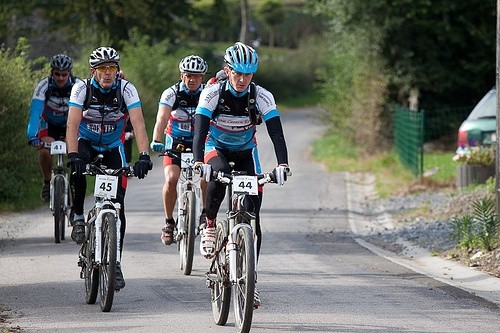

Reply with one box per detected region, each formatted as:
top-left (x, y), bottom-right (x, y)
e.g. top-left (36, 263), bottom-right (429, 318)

top-left (195, 164), bottom-right (292, 333)
top-left (153, 140), bottom-right (202, 275)
top-left (65, 154), bottom-right (153, 312)
top-left (41, 141), bottom-right (73, 244)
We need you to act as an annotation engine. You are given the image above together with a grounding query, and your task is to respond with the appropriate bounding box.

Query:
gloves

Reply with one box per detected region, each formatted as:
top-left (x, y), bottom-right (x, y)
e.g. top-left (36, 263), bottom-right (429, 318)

top-left (195, 162), bottom-right (212, 182)
top-left (66, 153), bottom-right (83, 173)
top-left (133, 156), bottom-right (153, 180)
top-left (32, 140), bottom-right (41, 147)
top-left (150, 141), bottom-right (164, 152)
top-left (270, 164), bottom-right (292, 186)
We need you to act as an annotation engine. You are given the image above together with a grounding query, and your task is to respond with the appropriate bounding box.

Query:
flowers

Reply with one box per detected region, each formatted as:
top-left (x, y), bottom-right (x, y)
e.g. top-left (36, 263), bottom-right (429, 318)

top-left (453, 139), bottom-right (496, 167)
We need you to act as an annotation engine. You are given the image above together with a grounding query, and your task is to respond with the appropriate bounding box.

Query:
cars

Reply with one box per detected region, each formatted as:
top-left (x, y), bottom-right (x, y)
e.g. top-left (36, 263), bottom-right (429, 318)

top-left (453, 84), bottom-right (497, 161)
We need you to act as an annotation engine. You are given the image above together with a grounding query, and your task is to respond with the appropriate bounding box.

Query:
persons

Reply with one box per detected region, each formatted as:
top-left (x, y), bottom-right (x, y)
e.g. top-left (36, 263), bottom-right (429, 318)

top-left (28, 46), bottom-right (152, 289)
top-left (149, 55), bottom-right (209, 245)
top-left (194, 42), bottom-right (291, 307)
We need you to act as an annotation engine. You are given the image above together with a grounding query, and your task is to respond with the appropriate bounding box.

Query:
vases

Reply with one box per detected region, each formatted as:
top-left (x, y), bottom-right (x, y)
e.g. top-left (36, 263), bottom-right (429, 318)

top-left (457, 164), bottom-right (496, 186)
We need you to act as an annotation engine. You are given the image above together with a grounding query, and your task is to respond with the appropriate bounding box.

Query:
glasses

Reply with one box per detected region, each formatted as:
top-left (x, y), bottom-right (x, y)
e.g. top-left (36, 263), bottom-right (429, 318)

top-left (232, 72), bottom-right (252, 77)
top-left (95, 66), bottom-right (118, 72)
top-left (186, 75), bottom-right (201, 79)
top-left (54, 72), bottom-right (68, 77)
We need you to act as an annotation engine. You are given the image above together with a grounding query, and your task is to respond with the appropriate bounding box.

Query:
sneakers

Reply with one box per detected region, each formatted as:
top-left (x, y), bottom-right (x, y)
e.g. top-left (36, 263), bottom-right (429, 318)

top-left (161, 221), bottom-right (175, 245)
top-left (113, 262), bottom-right (125, 290)
top-left (41, 179), bottom-right (50, 201)
top-left (200, 227), bottom-right (217, 259)
top-left (199, 214), bottom-right (207, 230)
top-left (71, 218), bottom-right (85, 243)
top-left (254, 288), bottom-right (261, 307)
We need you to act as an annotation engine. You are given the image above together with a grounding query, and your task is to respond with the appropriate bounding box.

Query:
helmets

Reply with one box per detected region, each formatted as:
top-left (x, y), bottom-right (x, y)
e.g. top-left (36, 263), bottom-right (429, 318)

top-left (50, 54), bottom-right (73, 72)
top-left (224, 42), bottom-right (259, 74)
top-left (178, 54), bottom-right (208, 75)
top-left (89, 46), bottom-right (120, 68)
